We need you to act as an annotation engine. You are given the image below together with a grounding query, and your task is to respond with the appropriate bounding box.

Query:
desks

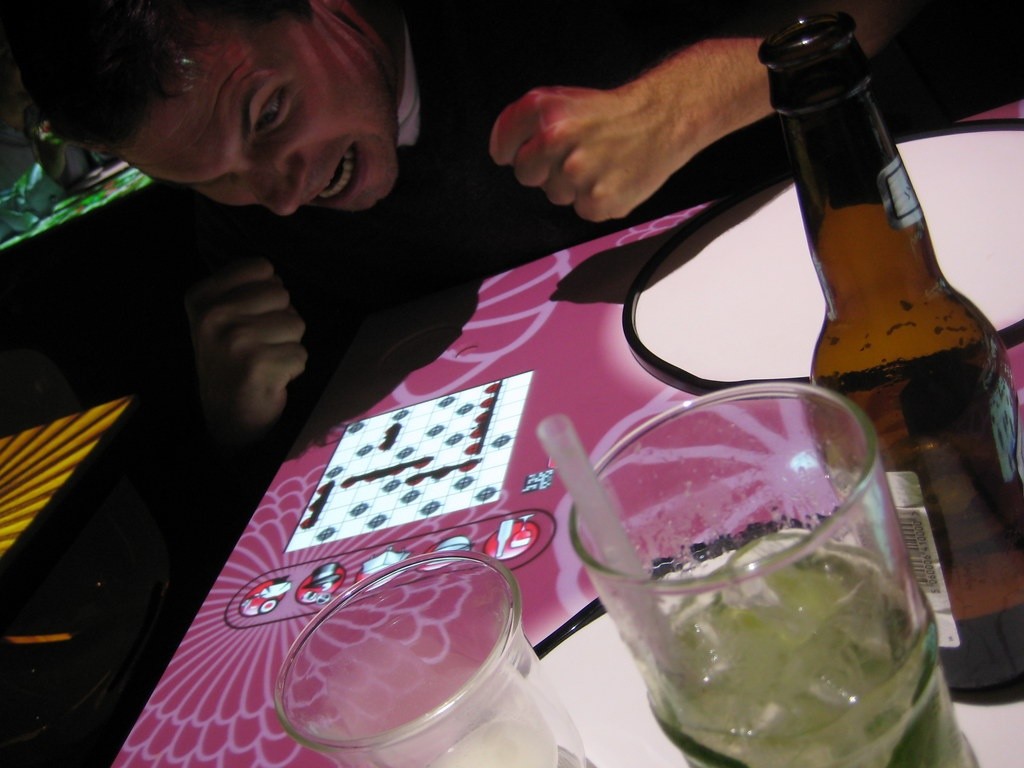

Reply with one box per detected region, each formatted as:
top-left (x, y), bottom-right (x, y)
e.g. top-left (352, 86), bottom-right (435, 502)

top-left (0, 383), bottom-right (177, 766)
top-left (0, 168), bottom-right (157, 410)
top-left (85, 94), bottom-right (1023, 768)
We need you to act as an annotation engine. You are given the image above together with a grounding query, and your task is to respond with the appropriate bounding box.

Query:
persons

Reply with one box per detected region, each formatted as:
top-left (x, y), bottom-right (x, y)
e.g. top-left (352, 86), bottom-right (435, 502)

top-left (0, 1), bottom-right (927, 450)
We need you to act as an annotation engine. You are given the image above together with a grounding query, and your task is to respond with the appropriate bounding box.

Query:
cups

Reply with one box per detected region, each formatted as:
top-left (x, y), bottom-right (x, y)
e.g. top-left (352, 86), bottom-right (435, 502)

top-left (568, 383), bottom-right (980, 768)
top-left (272, 550), bottom-right (587, 768)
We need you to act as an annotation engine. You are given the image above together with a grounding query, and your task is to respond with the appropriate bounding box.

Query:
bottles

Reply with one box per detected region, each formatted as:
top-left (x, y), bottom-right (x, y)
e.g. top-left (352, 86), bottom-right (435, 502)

top-left (756, 12), bottom-right (1024, 706)
